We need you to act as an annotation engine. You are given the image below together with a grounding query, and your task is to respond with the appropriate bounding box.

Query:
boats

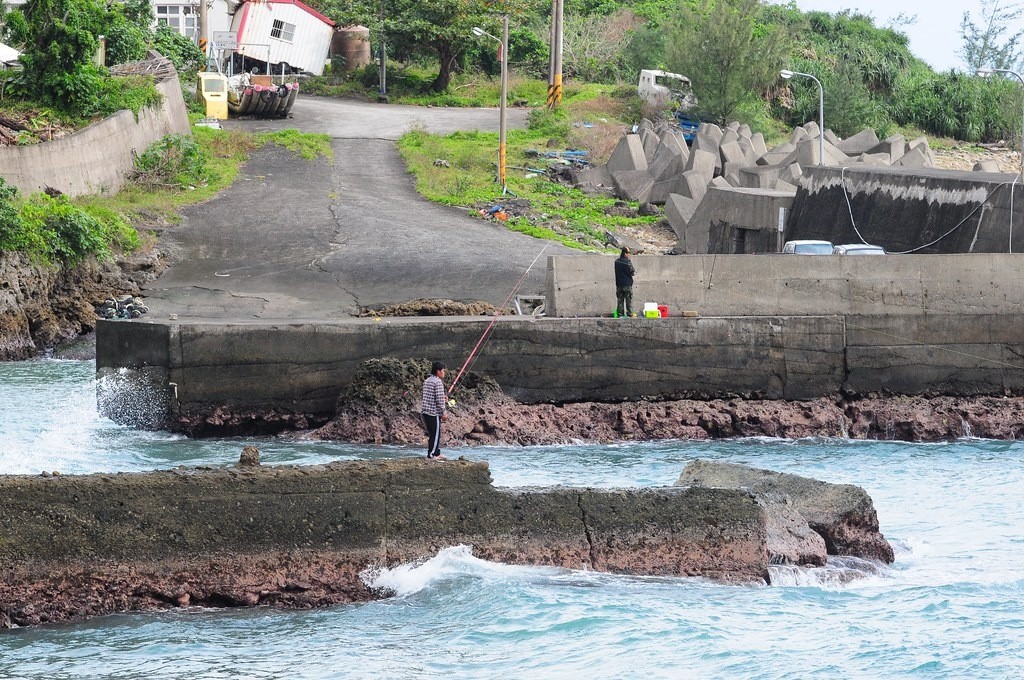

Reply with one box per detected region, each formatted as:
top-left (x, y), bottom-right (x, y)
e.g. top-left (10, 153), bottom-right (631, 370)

top-left (208, 42), bottom-right (299, 117)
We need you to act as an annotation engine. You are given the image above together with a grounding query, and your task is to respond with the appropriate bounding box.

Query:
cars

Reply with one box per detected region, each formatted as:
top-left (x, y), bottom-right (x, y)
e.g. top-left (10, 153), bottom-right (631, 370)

top-left (832, 244), bottom-right (888, 256)
top-left (783, 240), bottom-right (834, 254)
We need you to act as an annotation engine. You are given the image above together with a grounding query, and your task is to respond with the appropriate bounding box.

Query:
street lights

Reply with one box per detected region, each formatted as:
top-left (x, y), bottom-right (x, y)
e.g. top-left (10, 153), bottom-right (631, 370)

top-left (975, 68), bottom-right (1024, 183)
top-left (780, 69), bottom-right (825, 167)
top-left (472, 17), bottom-right (507, 187)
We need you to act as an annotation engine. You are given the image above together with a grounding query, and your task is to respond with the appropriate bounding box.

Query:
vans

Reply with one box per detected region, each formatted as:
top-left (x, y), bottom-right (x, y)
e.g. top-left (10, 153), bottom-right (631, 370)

top-left (639, 70), bottom-right (698, 112)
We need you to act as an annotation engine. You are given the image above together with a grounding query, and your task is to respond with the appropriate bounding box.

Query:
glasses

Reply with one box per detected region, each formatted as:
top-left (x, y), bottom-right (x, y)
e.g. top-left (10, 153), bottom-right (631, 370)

top-left (624, 252), bottom-right (632, 254)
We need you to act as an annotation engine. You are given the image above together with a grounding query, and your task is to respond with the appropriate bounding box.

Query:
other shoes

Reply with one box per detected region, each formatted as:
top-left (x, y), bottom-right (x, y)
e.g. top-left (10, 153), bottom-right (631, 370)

top-left (424, 456), bottom-right (444, 465)
top-left (434, 454), bottom-right (448, 461)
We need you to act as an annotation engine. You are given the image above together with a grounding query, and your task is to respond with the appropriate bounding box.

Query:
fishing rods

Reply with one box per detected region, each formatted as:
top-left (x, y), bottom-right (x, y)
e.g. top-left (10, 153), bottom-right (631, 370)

top-left (446, 241), bottom-right (554, 410)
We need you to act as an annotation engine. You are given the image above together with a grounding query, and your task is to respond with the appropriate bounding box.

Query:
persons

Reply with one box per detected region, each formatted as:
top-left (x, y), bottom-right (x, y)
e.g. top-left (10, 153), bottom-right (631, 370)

top-left (615, 247), bottom-right (637, 317)
top-left (423, 363), bottom-right (449, 454)
